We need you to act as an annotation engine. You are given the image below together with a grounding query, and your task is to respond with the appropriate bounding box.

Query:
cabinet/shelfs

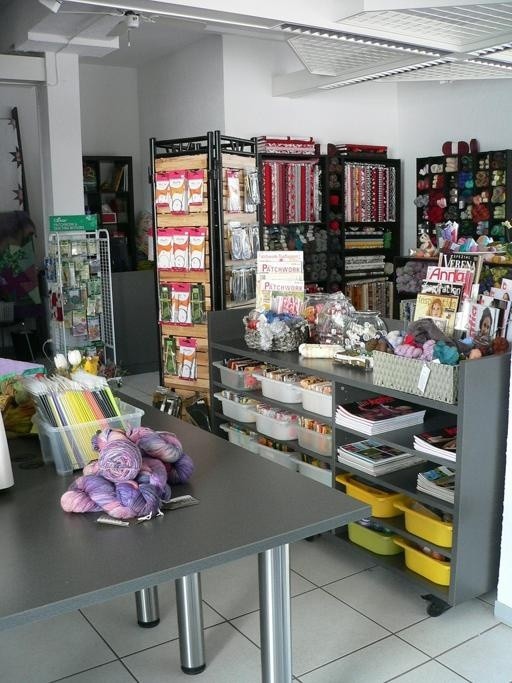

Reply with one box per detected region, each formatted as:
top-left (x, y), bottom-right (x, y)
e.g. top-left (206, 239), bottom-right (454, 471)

top-left (416, 150), bottom-right (512, 248)
top-left (327, 155), bottom-right (401, 320)
top-left (258, 153), bottom-right (342, 293)
top-left (150, 130), bottom-right (259, 431)
top-left (82, 155), bottom-right (136, 272)
top-left (208, 309), bottom-right (511, 617)
top-left (394, 258), bottom-right (512, 322)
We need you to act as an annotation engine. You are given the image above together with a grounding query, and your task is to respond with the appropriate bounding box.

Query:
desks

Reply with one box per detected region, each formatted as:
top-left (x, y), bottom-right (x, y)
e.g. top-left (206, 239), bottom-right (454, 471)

top-left (0, 387), bottom-right (373, 683)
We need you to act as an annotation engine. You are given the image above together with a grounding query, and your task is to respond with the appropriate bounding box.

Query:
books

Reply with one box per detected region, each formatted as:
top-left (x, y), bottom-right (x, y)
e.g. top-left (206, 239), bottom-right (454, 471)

top-left (254, 249), bottom-right (306, 317)
top-left (101, 164), bottom-right (128, 192)
top-left (22, 370), bottom-right (131, 470)
top-left (332, 392), bottom-right (456, 502)
top-left (408, 259), bottom-right (512, 352)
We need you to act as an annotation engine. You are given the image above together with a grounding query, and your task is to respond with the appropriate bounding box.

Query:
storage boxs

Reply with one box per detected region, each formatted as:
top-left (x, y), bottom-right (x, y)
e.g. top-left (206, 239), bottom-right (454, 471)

top-left (31, 397), bottom-right (145, 477)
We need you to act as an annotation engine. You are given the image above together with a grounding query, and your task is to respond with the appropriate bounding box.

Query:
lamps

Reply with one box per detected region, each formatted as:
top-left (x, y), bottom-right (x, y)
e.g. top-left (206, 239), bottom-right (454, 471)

top-left (38, 0), bottom-right (64, 14)
